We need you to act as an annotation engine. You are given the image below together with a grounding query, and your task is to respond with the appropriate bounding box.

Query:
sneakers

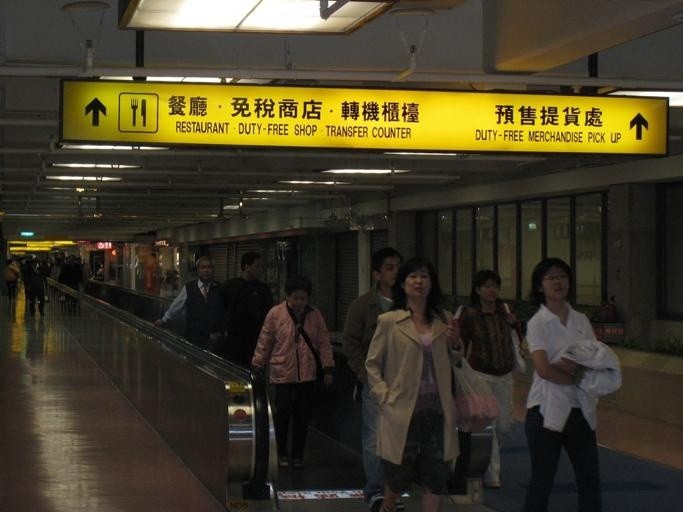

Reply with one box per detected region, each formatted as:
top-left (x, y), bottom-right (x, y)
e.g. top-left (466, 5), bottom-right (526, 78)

top-left (280, 456), bottom-right (303, 470)
top-left (369, 493), bottom-right (405, 512)
top-left (489, 481), bottom-right (500, 488)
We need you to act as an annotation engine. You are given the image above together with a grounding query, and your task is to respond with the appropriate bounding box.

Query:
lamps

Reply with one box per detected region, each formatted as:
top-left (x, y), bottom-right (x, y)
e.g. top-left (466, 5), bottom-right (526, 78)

top-left (114, 1), bottom-right (395, 36)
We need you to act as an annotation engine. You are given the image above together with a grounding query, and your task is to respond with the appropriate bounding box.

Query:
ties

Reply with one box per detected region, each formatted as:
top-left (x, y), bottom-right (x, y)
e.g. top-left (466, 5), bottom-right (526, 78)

top-left (202, 284), bottom-right (208, 305)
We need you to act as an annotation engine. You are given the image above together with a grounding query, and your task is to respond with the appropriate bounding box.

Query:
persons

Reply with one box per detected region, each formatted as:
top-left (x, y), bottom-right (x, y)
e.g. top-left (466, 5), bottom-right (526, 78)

top-left (459, 269), bottom-right (527, 488)
top-left (362, 257), bottom-right (466, 512)
top-left (342, 246), bottom-right (406, 512)
top-left (206, 252), bottom-right (275, 369)
top-left (3, 253), bottom-right (89, 316)
top-left (248, 274), bottom-right (337, 470)
top-left (521, 256), bottom-right (603, 512)
top-left (153, 254), bottom-right (222, 350)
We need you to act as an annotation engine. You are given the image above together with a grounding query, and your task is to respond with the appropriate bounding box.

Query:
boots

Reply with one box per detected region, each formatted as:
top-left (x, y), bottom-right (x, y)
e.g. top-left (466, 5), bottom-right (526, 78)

top-left (382, 485), bottom-right (401, 511)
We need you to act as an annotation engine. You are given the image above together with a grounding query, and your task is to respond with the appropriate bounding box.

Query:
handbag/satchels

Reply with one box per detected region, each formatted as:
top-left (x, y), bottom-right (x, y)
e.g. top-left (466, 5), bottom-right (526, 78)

top-left (509, 327), bottom-right (525, 373)
